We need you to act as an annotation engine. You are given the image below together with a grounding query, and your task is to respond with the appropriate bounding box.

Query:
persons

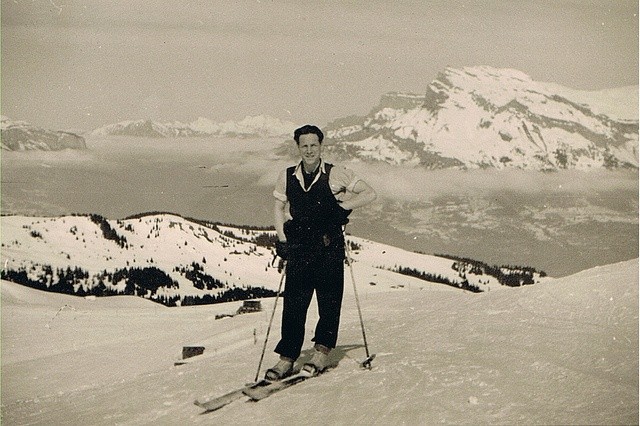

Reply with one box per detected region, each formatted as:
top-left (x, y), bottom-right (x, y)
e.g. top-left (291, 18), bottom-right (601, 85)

top-left (266, 124), bottom-right (377, 383)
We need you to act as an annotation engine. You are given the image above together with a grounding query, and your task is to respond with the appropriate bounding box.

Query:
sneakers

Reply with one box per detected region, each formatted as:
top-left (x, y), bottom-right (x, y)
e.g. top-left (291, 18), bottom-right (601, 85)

top-left (267, 356), bottom-right (295, 378)
top-left (303, 344), bottom-right (330, 374)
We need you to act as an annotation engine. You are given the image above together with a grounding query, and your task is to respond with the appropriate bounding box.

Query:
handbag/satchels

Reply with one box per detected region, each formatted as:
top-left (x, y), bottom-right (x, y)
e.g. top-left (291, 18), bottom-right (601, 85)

top-left (275, 219), bottom-right (316, 261)
